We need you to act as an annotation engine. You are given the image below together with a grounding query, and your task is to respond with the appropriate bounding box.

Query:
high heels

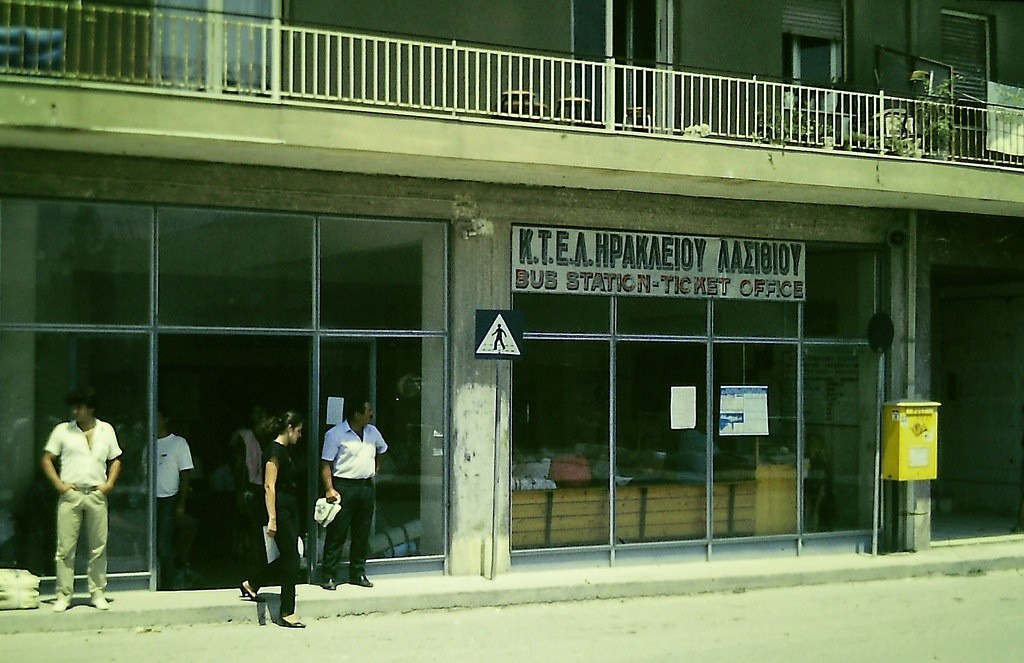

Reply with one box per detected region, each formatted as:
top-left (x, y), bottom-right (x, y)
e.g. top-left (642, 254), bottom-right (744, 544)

top-left (240, 581), bottom-right (264, 602)
top-left (278, 614), bottom-right (306, 628)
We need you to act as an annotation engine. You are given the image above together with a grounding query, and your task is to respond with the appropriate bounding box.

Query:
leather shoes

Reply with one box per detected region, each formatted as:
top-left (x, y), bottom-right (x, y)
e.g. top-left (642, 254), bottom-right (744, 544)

top-left (52, 590), bottom-right (71, 612)
top-left (350, 574), bottom-right (373, 587)
top-left (91, 590), bottom-right (110, 609)
top-left (320, 577), bottom-right (336, 590)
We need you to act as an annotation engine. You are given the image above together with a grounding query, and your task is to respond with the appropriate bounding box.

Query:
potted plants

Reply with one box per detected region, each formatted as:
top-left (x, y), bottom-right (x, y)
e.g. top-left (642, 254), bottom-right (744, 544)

top-left (818, 124), bottom-right (834, 151)
top-left (917, 78), bottom-right (963, 160)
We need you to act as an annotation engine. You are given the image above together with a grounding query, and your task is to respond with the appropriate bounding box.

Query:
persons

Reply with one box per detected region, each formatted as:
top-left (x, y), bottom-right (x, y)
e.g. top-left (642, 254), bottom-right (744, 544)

top-left (227, 407), bottom-right (307, 628)
top-left (139, 408), bottom-right (194, 588)
top-left (40, 386), bottom-right (121, 611)
top-left (319, 399), bottom-right (388, 591)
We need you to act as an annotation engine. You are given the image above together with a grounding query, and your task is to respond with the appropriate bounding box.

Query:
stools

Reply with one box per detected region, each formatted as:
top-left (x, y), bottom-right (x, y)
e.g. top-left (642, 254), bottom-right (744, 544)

top-left (555, 97), bottom-right (596, 129)
top-left (623, 106), bottom-right (651, 133)
top-left (496, 91), bottom-right (548, 123)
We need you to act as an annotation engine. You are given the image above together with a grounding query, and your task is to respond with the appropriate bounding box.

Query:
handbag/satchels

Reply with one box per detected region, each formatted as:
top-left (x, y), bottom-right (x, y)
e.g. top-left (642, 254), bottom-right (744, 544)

top-left (314, 496), bottom-right (342, 528)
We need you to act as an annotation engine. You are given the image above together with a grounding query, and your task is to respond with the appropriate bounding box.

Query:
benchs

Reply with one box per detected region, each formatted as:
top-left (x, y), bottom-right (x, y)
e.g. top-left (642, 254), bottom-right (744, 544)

top-left (511, 478), bottom-right (758, 549)
